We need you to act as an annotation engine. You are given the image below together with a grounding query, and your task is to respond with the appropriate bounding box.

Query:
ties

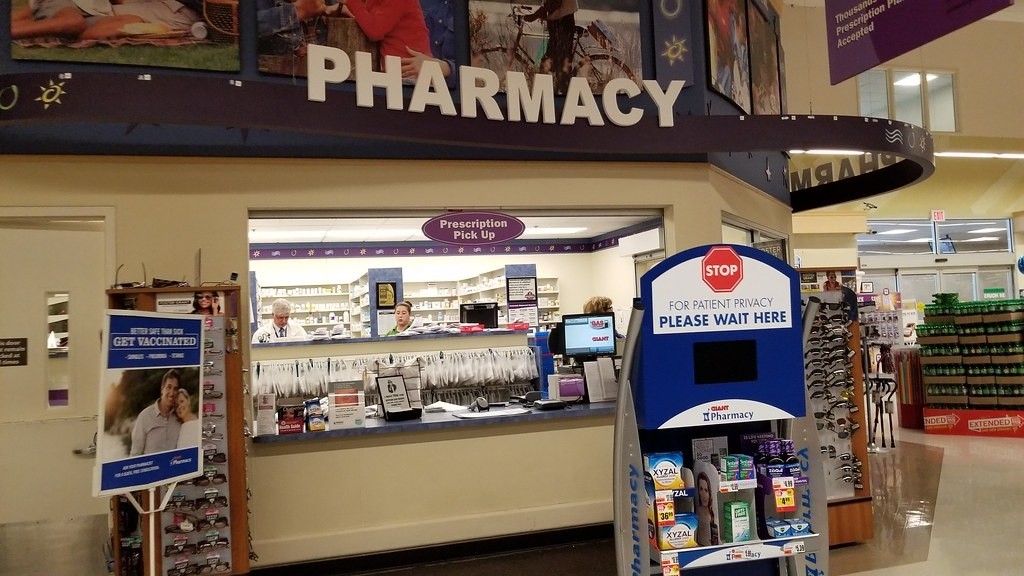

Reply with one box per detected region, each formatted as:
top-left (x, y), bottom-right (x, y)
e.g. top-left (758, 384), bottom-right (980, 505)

top-left (279, 327), bottom-right (284, 337)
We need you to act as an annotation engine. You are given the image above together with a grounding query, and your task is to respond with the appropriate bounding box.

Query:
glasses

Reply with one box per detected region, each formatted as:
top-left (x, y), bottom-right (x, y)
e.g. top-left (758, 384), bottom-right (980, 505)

top-left (193, 294), bottom-right (212, 300)
top-left (607, 305), bottom-right (613, 310)
top-left (200, 280), bottom-right (238, 286)
top-left (205, 318), bottom-right (213, 327)
top-left (805, 301), bottom-right (860, 439)
top-left (198, 562), bottom-right (230, 575)
top-left (164, 544), bottom-right (197, 557)
top-left (162, 500), bottom-right (196, 512)
top-left (821, 445), bottom-right (836, 454)
top-left (196, 516), bottom-right (229, 532)
top-left (164, 523), bottom-right (195, 534)
top-left (165, 338), bottom-right (228, 488)
top-left (834, 453), bottom-right (863, 490)
top-left (196, 496), bottom-right (228, 511)
top-left (197, 537), bottom-right (230, 554)
top-left (167, 563), bottom-right (198, 576)
top-left (226, 316), bottom-right (259, 562)
top-left (111, 262), bottom-right (147, 289)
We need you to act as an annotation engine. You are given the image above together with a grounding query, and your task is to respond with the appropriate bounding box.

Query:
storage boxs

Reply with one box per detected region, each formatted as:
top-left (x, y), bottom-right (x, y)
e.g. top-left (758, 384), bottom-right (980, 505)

top-left (279, 390), bottom-right (366, 435)
top-left (642, 451), bottom-right (810, 551)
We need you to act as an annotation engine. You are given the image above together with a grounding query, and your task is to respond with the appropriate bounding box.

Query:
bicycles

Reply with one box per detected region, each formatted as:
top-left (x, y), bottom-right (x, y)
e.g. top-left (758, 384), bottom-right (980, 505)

top-left (473, 5), bottom-right (636, 92)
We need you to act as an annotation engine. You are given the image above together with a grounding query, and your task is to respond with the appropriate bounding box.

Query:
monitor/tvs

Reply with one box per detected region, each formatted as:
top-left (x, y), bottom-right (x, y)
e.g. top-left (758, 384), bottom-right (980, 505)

top-left (460, 303), bottom-right (498, 328)
top-left (562, 312), bottom-right (617, 361)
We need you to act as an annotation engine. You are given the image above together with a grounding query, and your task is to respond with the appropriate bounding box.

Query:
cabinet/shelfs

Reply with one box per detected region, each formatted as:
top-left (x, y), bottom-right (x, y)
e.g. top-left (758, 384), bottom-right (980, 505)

top-left (247, 264), bottom-right (562, 339)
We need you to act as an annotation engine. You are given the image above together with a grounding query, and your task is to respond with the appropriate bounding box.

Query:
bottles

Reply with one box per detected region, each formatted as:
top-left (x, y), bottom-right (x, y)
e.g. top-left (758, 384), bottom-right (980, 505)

top-left (258, 274), bottom-right (507, 325)
top-left (915, 299), bottom-right (1024, 410)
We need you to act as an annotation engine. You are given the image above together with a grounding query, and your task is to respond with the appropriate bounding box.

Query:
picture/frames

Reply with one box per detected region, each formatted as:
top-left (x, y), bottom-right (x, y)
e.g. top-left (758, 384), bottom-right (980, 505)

top-left (861, 282), bottom-right (873, 293)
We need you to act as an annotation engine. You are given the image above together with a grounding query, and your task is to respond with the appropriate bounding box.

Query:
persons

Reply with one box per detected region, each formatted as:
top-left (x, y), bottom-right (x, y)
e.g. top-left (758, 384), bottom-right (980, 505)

top-left (695, 472), bottom-right (714, 546)
top-left (583, 296), bottom-right (625, 338)
top-left (252, 299), bottom-right (308, 343)
top-left (129, 370), bottom-right (183, 457)
top-left (189, 290), bottom-right (224, 315)
top-left (10, 0), bottom-right (578, 95)
top-left (823, 270), bottom-right (843, 291)
top-left (173, 388), bottom-right (200, 449)
top-left (282, 407), bottom-right (297, 419)
top-left (383, 301), bottom-right (413, 337)
